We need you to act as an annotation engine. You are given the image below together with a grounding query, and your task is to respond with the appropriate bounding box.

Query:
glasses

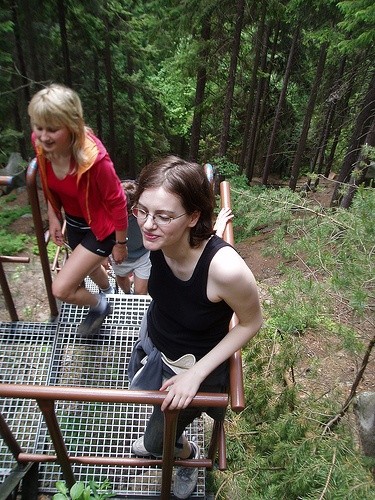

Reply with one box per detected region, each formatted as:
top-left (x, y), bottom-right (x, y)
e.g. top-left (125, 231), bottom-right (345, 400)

top-left (131, 203), bottom-right (193, 225)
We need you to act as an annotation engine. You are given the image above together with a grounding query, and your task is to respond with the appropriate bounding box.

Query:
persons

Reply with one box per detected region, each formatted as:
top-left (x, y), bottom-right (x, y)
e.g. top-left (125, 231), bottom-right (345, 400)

top-left (101, 180), bottom-right (152, 295)
top-left (129, 156), bottom-right (264, 499)
top-left (27, 82), bottom-right (128, 336)
top-left (213, 207), bottom-right (234, 239)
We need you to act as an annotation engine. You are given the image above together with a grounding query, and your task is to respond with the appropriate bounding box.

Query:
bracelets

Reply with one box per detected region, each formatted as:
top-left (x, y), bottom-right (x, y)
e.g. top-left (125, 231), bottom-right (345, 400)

top-left (116, 237), bottom-right (128, 244)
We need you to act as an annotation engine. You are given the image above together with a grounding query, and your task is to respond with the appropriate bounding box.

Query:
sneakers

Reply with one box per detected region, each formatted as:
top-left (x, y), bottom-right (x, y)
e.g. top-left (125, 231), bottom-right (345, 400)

top-left (76, 303), bottom-right (111, 337)
top-left (108, 287), bottom-right (119, 302)
top-left (132, 435), bottom-right (151, 456)
top-left (173, 441), bottom-right (200, 499)
